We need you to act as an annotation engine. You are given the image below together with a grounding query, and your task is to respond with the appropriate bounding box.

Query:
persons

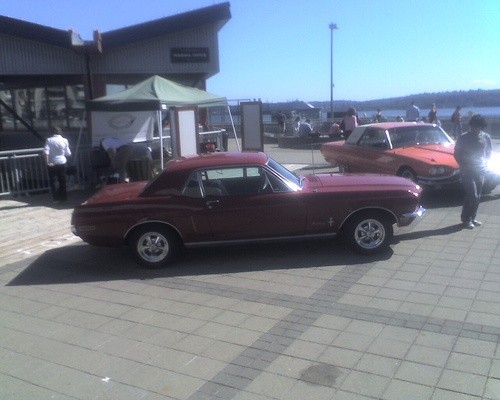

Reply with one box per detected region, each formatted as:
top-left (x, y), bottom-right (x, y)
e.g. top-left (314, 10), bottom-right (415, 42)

top-left (428, 102), bottom-right (441, 127)
top-left (406, 102), bottom-right (421, 121)
top-left (451, 105), bottom-right (464, 139)
top-left (285, 113), bottom-right (298, 136)
top-left (100, 136), bottom-right (129, 184)
top-left (295, 116), bottom-right (300, 132)
top-left (299, 118), bottom-right (313, 137)
top-left (329, 120), bottom-right (342, 137)
top-left (454, 114), bottom-right (493, 229)
top-left (376, 108), bottom-right (381, 122)
top-left (396, 116), bottom-right (406, 133)
top-left (345, 107), bottom-right (358, 140)
top-left (43, 126), bottom-right (71, 205)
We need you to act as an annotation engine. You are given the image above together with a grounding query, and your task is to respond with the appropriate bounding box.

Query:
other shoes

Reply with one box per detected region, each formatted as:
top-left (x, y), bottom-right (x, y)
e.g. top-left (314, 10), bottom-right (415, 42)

top-left (472, 219), bottom-right (481, 226)
top-left (461, 216), bottom-right (474, 228)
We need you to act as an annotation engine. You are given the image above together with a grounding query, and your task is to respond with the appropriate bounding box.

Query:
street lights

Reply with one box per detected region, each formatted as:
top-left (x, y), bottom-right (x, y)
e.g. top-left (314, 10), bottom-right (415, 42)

top-left (329, 22), bottom-right (337, 124)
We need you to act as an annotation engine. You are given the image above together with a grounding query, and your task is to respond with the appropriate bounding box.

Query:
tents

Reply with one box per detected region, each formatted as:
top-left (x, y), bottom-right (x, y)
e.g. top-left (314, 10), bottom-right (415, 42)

top-left (71, 75), bottom-right (241, 167)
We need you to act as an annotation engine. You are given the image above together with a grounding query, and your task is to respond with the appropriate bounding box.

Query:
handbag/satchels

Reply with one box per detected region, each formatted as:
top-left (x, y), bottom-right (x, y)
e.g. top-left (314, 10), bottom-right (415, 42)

top-left (66, 165), bottom-right (77, 175)
top-left (340, 116), bottom-right (345, 130)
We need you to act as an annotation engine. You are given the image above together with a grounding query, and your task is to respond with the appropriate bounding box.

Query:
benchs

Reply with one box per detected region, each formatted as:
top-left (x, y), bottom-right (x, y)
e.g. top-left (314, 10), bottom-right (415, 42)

top-left (187, 172), bottom-right (222, 196)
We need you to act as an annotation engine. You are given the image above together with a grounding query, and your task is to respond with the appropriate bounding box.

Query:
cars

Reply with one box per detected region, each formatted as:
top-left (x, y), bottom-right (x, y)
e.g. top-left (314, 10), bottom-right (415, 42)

top-left (319, 121), bottom-right (498, 195)
top-left (69, 152), bottom-right (422, 273)
